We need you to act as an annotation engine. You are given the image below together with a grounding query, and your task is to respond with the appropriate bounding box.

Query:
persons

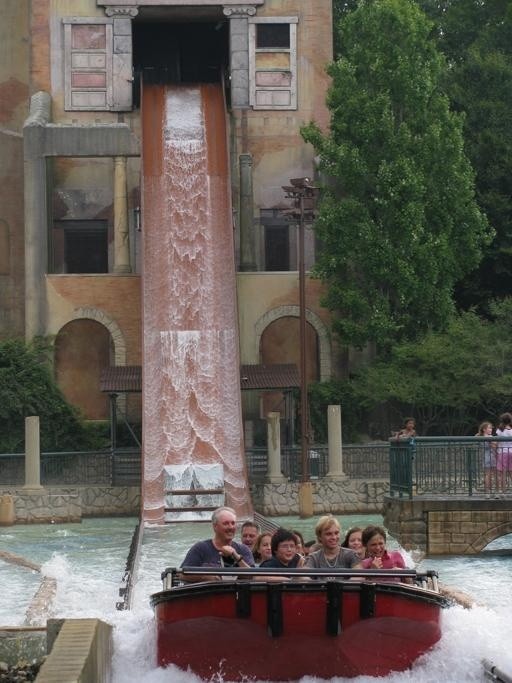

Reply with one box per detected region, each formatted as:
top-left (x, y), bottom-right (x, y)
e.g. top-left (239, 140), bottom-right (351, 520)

top-left (475, 422), bottom-right (496, 497)
top-left (177, 507), bottom-right (415, 587)
top-left (494, 412), bottom-right (512, 494)
top-left (395, 418), bottom-right (416, 460)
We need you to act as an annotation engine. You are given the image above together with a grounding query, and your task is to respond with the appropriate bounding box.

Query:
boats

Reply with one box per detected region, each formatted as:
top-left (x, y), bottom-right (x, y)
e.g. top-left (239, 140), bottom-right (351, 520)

top-left (149, 565), bottom-right (446, 681)
